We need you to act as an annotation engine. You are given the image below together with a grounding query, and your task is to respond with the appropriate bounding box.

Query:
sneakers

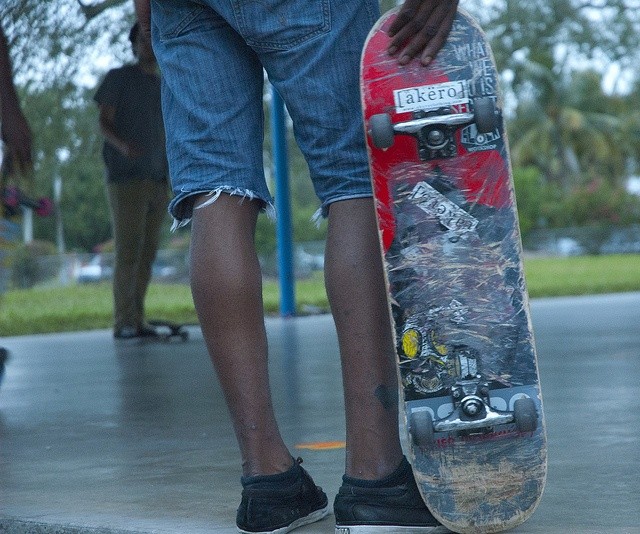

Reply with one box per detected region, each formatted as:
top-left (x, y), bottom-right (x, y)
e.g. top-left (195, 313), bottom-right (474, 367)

top-left (237, 457), bottom-right (331, 532)
top-left (137, 324), bottom-right (170, 338)
top-left (333, 466), bottom-right (442, 532)
top-left (115, 328), bottom-right (147, 346)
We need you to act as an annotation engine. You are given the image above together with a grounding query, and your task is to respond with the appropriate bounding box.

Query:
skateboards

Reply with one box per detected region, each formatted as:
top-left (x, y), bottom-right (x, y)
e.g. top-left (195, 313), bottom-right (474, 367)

top-left (360, 2), bottom-right (548, 533)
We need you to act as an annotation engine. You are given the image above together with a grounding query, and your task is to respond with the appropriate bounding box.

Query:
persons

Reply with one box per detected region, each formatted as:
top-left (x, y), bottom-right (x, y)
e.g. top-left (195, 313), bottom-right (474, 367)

top-left (134, 2), bottom-right (462, 534)
top-left (0, 27), bottom-right (27, 379)
top-left (92, 21), bottom-right (173, 339)
